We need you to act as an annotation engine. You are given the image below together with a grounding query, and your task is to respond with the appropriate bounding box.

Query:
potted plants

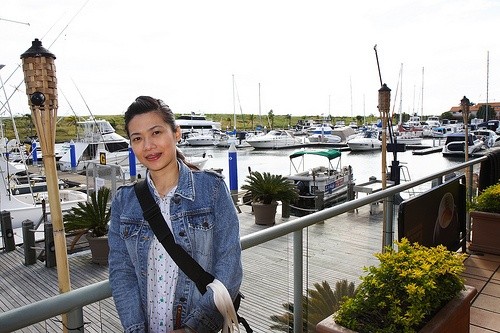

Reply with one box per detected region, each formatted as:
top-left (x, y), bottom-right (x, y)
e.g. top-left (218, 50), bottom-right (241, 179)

top-left (63, 186), bottom-right (111, 264)
top-left (242, 171), bottom-right (300, 226)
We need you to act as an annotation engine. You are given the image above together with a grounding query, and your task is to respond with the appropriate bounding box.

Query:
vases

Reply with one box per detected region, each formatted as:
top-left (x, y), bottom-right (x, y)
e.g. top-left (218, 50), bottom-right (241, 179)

top-left (468, 211), bottom-right (500, 255)
top-left (315, 284), bottom-right (477, 333)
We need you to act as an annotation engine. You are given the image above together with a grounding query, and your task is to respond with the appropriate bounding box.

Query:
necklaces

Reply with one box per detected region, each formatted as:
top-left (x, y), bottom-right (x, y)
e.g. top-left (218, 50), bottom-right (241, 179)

top-left (158, 178), bottom-right (178, 197)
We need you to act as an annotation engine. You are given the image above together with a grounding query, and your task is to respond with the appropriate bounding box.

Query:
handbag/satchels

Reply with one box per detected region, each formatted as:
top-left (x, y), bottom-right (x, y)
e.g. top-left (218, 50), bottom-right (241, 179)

top-left (197, 271), bottom-right (254, 332)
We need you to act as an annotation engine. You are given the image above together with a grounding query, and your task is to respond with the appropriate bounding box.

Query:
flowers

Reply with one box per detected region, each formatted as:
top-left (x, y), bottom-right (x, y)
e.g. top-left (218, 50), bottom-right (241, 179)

top-left (466, 182), bottom-right (500, 213)
top-left (330, 237), bottom-right (470, 333)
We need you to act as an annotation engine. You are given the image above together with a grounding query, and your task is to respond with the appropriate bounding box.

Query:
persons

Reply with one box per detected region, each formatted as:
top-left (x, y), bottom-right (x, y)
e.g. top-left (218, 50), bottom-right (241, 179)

top-left (109, 96), bottom-right (243, 333)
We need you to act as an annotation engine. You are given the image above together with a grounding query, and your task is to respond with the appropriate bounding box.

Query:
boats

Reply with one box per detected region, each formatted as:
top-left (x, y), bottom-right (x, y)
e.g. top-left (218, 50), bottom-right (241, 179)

top-left (0, 0), bottom-right (214, 233)
top-left (286, 148), bottom-right (356, 219)
top-left (174, 63), bottom-right (500, 157)
